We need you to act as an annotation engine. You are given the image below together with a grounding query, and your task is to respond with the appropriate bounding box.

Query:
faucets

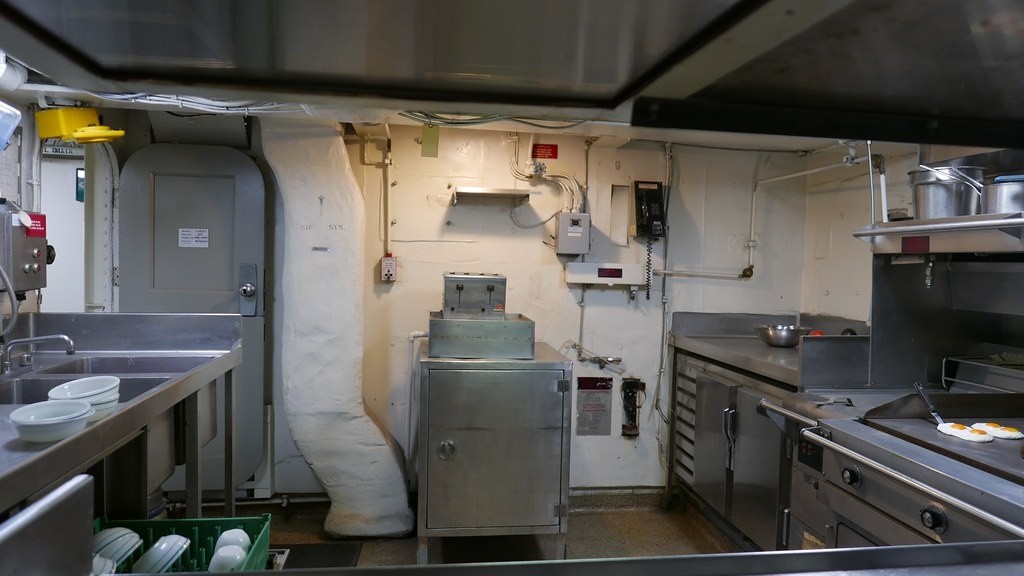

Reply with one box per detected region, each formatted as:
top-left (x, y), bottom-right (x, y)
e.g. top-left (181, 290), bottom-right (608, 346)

top-left (5, 334), bottom-right (75, 361)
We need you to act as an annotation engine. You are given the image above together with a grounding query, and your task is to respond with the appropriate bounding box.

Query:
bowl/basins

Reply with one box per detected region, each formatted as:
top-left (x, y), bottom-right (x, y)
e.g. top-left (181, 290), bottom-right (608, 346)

top-left (132, 534), bottom-right (191, 573)
top-left (48, 374), bottom-right (121, 423)
top-left (11, 399), bottom-right (95, 443)
top-left (90, 525), bottom-right (142, 576)
top-left (753, 324), bottom-right (814, 347)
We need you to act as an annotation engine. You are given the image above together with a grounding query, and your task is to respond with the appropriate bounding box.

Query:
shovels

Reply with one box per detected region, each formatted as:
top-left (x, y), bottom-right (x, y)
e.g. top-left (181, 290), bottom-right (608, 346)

top-left (914, 378), bottom-right (946, 424)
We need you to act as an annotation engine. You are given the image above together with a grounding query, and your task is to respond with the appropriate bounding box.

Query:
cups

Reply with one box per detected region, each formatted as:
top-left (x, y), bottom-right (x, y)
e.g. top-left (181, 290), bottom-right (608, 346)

top-left (207, 528), bottom-right (251, 572)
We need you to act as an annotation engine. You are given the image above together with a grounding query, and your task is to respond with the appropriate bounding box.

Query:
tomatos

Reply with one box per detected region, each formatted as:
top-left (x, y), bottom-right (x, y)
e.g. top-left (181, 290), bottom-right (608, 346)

top-left (811, 331), bottom-right (823, 335)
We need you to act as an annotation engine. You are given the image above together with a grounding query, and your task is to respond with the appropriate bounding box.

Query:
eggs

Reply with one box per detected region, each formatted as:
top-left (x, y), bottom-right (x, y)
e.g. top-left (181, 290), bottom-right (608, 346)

top-left (971, 422), bottom-right (1024, 438)
top-left (936, 423), bottom-right (993, 442)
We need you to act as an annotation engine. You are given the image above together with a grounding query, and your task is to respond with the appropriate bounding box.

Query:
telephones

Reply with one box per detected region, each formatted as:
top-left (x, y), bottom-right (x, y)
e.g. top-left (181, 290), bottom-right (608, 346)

top-left (634, 180), bottom-right (666, 239)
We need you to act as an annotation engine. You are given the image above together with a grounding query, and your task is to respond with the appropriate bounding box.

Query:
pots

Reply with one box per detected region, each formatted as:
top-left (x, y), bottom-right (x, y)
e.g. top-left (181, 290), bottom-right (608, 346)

top-left (920, 164), bottom-right (1024, 214)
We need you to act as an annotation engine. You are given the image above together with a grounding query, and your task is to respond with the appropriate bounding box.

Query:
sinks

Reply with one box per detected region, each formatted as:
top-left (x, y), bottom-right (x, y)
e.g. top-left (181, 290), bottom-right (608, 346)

top-left (38, 355), bottom-right (214, 376)
top-left (0, 377), bottom-right (174, 404)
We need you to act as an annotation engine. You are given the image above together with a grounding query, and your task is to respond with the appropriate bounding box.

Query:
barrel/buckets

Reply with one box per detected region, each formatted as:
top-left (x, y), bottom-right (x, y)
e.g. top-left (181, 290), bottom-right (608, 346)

top-left (907, 165), bottom-right (987, 219)
